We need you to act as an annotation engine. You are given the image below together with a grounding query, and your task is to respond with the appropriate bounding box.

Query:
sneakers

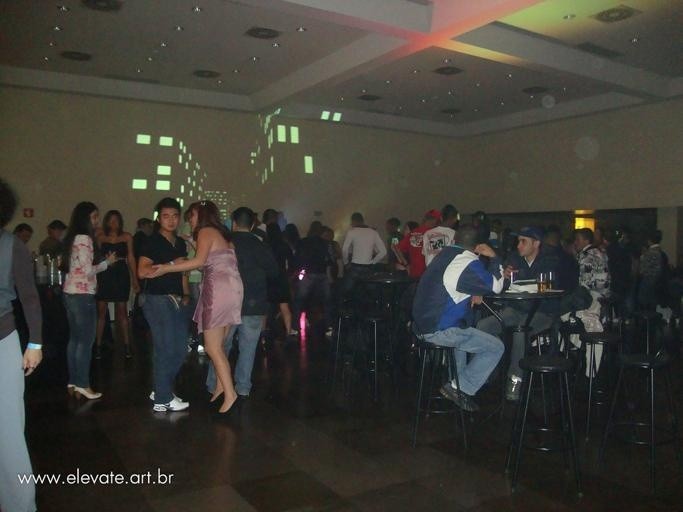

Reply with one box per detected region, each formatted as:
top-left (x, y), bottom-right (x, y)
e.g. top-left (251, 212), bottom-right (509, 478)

top-left (149, 390), bottom-right (190, 413)
top-left (439, 381), bottom-right (481, 414)
top-left (505, 375), bottom-right (524, 402)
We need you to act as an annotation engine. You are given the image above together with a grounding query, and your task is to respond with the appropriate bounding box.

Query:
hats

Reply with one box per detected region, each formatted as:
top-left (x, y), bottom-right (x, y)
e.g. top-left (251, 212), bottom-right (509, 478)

top-left (425, 209), bottom-right (442, 222)
top-left (510, 224), bottom-right (546, 244)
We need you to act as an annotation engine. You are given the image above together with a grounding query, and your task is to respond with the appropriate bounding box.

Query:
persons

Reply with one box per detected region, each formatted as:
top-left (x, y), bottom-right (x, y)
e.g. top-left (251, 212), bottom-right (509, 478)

top-left (0, 184), bottom-right (45, 512)
top-left (13, 198), bottom-right (683, 420)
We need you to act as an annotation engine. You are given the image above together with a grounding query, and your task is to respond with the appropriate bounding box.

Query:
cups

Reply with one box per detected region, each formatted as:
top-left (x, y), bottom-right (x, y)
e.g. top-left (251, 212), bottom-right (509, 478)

top-left (509, 267), bottom-right (520, 283)
top-left (537, 273), bottom-right (547, 292)
top-left (548, 270), bottom-right (556, 289)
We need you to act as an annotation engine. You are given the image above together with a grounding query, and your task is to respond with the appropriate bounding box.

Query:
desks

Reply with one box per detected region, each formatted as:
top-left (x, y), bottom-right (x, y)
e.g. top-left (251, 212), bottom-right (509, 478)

top-left (473, 287), bottom-right (577, 405)
top-left (352, 275), bottom-right (419, 362)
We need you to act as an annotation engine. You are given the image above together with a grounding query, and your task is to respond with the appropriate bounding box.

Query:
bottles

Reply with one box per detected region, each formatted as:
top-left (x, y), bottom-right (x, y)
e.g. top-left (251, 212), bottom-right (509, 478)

top-left (57, 257), bottom-right (65, 285)
top-left (36, 250), bottom-right (48, 283)
top-left (49, 250), bottom-right (59, 286)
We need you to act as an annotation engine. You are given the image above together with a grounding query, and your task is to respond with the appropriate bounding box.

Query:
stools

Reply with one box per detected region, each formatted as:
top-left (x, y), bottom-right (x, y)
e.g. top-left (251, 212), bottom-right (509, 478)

top-left (598, 353), bottom-right (682, 497)
top-left (325, 296), bottom-right (366, 397)
top-left (520, 327), bottom-right (570, 433)
top-left (617, 310), bottom-right (673, 420)
top-left (569, 331), bottom-right (645, 447)
top-left (413, 341), bottom-right (470, 449)
top-left (352, 312), bottom-right (402, 402)
top-left (506, 355), bottom-right (583, 500)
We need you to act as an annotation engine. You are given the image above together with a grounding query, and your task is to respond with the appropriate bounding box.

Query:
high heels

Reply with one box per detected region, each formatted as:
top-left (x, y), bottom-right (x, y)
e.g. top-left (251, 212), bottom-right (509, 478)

top-left (283, 329), bottom-right (299, 339)
top-left (205, 391), bottom-right (225, 409)
top-left (123, 342), bottom-right (134, 360)
top-left (211, 395), bottom-right (242, 419)
top-left (66, 384), bottom-right (103, 400)
top-left (94, 344), bottom-right (106, 361)
